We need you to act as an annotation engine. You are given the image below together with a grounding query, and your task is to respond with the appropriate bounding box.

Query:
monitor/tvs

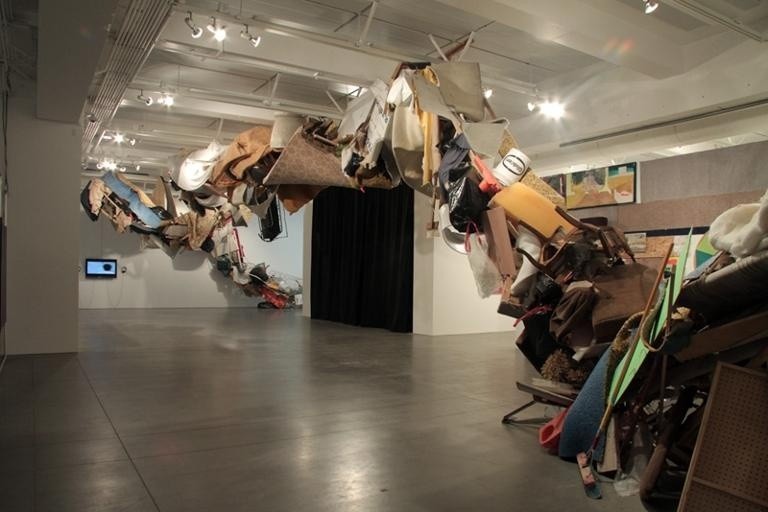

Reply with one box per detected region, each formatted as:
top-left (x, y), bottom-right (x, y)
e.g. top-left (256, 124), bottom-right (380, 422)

top-left (85, 258), bottom-right (117, 278)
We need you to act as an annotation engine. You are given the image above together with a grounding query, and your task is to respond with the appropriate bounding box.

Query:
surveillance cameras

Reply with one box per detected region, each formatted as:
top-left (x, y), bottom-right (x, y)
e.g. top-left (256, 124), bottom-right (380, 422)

top-left (88, 115), bottom-right (98, 122)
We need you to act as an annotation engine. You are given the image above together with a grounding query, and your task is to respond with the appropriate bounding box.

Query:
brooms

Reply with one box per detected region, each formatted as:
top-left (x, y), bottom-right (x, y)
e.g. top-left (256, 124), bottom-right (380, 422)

top-left (577, 243), bottom-right (674, 500)
top-left (641, 380), bottom-right (695, 498)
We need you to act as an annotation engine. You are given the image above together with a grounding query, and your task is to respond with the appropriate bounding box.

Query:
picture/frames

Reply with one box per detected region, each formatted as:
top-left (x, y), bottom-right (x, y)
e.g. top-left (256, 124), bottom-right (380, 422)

top-left (543, 161), bottom-right (638, 212)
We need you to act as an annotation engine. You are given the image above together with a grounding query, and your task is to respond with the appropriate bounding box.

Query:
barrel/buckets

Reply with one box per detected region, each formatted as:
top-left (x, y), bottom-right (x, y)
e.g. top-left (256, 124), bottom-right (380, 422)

top-left (493, 148), bottom-right (531, 188)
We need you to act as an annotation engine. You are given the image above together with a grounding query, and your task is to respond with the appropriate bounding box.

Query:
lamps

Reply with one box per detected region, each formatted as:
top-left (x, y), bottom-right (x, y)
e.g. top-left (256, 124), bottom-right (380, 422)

top-left (182, 10), bottom-right (263, 49)
top-left (135, 89), bottom-right (154, 106)
top-left (642, 0), bottom-right (659, 14)
